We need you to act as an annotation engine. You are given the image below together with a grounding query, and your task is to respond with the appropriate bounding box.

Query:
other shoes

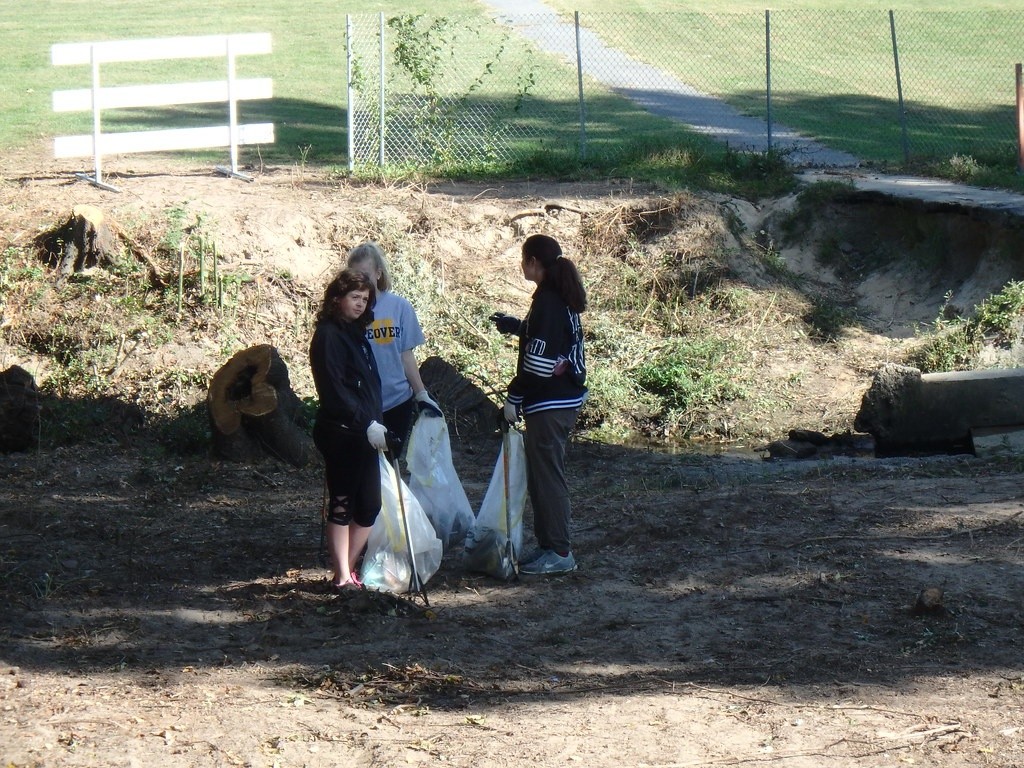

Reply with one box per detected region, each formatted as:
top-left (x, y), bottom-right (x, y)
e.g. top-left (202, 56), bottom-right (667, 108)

top-left (331, 571), bottom-right (364, 591)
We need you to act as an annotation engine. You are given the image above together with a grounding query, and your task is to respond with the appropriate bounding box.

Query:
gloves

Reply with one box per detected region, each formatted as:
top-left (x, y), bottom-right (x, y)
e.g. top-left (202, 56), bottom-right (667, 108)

top-left (415, 391), bottom-right (445, 418)
top-left (503, 400), bottom-right (522, 426)
top-left (488, 311), bottom-right (522, 335)
top-left (366, 419), bottom-right (389, 451)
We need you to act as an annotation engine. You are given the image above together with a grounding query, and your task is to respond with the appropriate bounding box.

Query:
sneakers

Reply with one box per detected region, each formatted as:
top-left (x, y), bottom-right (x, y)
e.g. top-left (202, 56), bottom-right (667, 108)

top-left (519, 549), bottom-right (578, 574)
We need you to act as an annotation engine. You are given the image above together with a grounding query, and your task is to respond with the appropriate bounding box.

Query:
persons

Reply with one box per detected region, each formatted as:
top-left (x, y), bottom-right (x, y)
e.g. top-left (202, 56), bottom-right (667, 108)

top-left (487, 234), bottom-right (592, 575)
top-left (347, 242), bottom-right (445, 472)
top-left (307, 268), bottom-right (389, 589)
